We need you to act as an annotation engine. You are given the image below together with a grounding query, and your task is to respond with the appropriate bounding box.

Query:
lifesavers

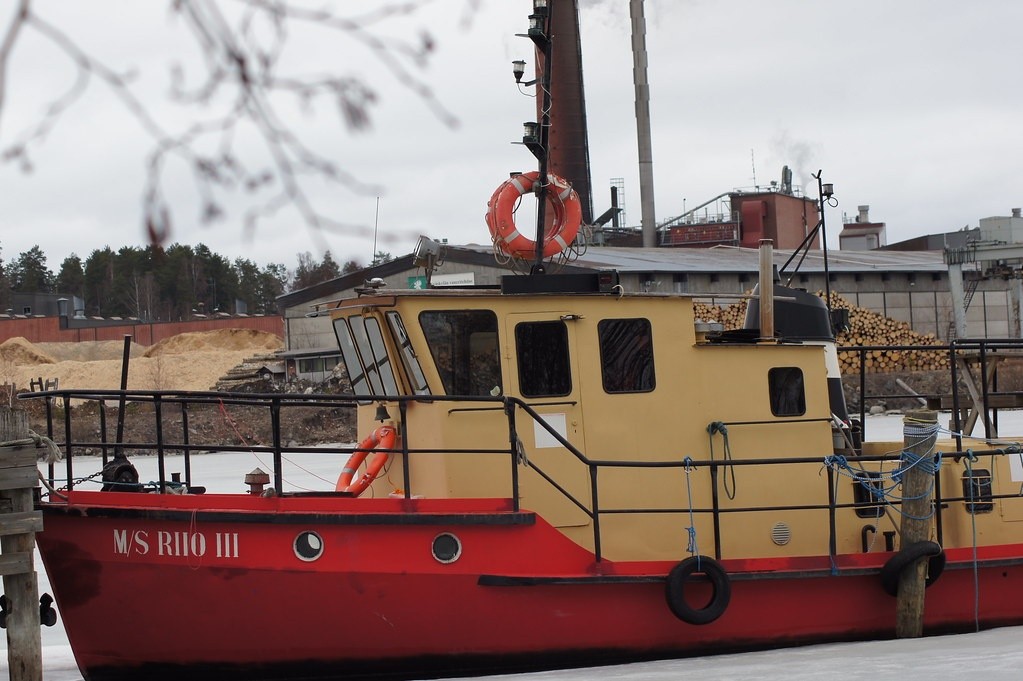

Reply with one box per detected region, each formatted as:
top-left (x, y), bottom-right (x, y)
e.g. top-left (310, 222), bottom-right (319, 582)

top-left (666, 555), bottom-right (732, 625)
top-left (487, 171), bottom-right (582, 259)
top-left (880, 543), bottom-right (946, 596)
top-left (336, 425), bottom-right (395, 497)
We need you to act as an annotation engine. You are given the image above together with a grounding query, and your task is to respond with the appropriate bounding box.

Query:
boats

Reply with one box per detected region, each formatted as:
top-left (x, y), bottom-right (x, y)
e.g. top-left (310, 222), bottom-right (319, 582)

top-left (29, 0), bottom-right (1023, 681)
